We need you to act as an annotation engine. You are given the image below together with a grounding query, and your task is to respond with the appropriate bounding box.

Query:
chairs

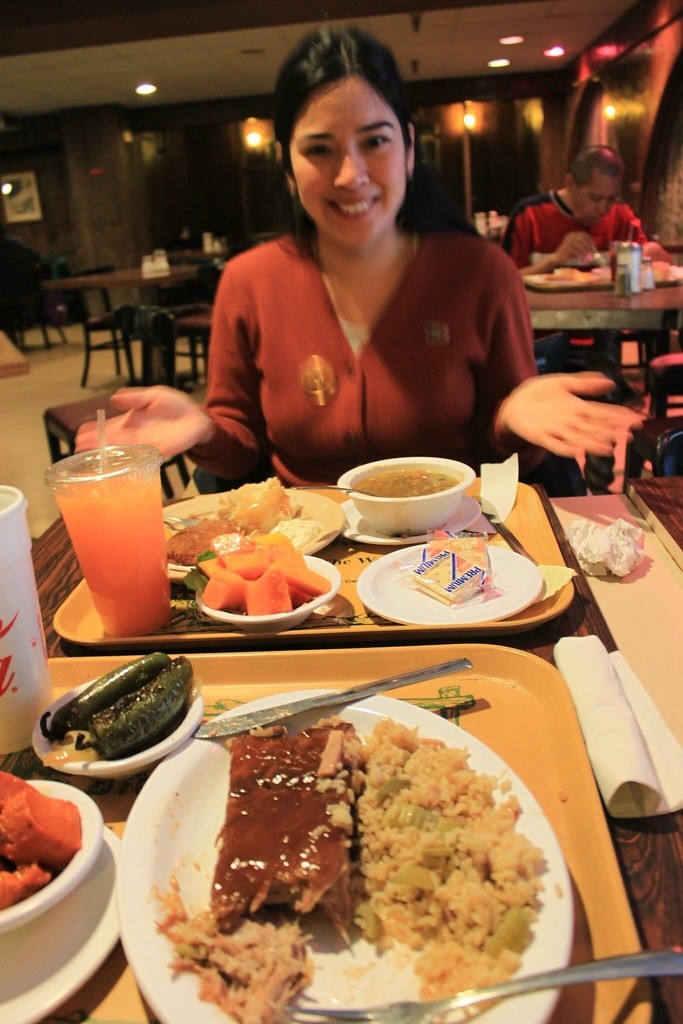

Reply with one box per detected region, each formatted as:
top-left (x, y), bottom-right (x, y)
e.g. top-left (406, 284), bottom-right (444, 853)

top-left (1, 238), bottom-right (683, 497)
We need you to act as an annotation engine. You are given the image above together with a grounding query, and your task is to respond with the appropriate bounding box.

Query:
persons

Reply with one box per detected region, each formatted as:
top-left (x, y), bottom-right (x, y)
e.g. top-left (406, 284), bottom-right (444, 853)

top-left (502, 145), bottom-right (648, 276)
top-left (75, 27), bottom-right (643, 488)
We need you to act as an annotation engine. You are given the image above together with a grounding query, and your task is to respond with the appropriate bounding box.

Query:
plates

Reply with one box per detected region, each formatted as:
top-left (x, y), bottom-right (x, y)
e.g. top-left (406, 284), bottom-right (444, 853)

top-left (339, 493), bottom-right (482, 546)
top-left (118, 688), bottom-right (573, 1024)
top-left (160, 490), bottom-right (346, 582)
top-left (655, 276), bottom-right (683, 287)
top-left (524, 273), bottom-right (614, 293)
top-left (0, 826), bottom-right (124, 1024)
top-left (356, 544), bottom-right (544, 625)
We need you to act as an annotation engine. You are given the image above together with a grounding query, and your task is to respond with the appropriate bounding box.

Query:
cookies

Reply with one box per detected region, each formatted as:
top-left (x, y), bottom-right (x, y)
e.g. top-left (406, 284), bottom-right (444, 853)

top-left (412, 537), bottom-right (489, 604)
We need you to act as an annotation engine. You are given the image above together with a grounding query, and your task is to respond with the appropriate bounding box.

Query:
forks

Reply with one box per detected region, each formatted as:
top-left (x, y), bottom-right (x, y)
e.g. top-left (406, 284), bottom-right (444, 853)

top-left (280, 948), bottom-right (683, 1024)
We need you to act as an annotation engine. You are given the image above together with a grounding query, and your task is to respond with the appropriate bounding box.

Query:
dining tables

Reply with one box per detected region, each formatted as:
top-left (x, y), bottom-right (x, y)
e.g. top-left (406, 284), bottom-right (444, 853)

top-left (522, 268), bottom-right (683, 419)
top-left (2, 475), bottom-right (683, 1024)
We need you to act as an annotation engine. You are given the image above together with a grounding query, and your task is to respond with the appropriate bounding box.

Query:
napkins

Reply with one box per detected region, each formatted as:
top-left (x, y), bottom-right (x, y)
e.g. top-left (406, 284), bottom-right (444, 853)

top-left (480, 453), bottom-right (519, 522)
top-left (554, 634), bottom-right (682, 818)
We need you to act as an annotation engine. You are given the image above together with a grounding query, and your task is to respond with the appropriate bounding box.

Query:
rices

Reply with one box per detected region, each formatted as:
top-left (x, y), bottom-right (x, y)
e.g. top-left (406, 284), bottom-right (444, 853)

top-left (314, 715), bottom-right (559, 1004)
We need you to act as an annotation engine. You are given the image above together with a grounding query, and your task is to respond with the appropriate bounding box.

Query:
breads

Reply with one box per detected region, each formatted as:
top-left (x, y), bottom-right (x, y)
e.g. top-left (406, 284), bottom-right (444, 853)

top-left (219, 476), bottom-right (292, 532)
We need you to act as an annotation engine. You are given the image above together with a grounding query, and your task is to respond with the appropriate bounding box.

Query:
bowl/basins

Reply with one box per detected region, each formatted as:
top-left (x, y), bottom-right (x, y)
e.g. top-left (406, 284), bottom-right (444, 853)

top-left (32, 675), bottom-right (205, 779)
top-left (0, 779), bottom-right (104, 932)
top-left (195, 556), bottom-right (342, 632)
top-left (336, 457), bottom-right (477, 536)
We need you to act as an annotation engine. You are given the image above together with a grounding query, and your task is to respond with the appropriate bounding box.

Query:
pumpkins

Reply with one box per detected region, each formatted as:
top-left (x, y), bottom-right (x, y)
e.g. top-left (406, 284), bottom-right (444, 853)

top-left (197, 530), bottom-right (333, 616)
top-left (0, 771), bottom-right (81, 909)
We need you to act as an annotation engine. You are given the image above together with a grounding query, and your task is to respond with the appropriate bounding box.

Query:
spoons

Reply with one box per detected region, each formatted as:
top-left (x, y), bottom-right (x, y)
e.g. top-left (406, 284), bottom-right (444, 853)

top-left (593, 251), bottom-right (606, 268)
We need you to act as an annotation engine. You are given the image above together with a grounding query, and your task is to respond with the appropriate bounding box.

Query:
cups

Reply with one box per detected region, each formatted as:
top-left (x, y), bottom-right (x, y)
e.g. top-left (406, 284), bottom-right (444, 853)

top-left (0, 485), bottom-right (54, 755)
top-left (44, 445), bottom-right (173, 637)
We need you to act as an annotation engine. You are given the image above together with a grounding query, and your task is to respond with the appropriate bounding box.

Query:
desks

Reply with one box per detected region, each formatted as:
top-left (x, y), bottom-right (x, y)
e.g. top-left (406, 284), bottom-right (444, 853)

top-left (40, 265), bottom-right (202, 387)
top-left (169, 247), bottom-right (227, 261)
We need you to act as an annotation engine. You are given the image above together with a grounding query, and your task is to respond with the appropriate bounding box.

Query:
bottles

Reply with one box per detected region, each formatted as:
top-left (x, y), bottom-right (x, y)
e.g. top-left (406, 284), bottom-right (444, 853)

top-left (474, 211), bottom-right (501, 240)
top-left (154, 249), bottom-right (169, 278)
top-left (202, 233), bottom-right (221, 253)
top-left (614, 241), bottom-right (655, 297)
top-left (141, 255), bottom-right (153, 279)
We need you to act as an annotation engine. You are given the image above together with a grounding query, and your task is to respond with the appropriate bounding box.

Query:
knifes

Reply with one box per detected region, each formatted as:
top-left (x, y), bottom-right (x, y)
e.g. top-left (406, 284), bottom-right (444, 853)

top-left (163, 514), bottom-right (199, 527)
top-left (191, 658), bottom-right (473, 740)
top-left (473, 495), bottom-right (538, 566)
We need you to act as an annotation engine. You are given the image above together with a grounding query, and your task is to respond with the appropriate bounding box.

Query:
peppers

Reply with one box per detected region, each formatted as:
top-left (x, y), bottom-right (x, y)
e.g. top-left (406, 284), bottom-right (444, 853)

top-left (39, 651), bottom-right (194, 758)
